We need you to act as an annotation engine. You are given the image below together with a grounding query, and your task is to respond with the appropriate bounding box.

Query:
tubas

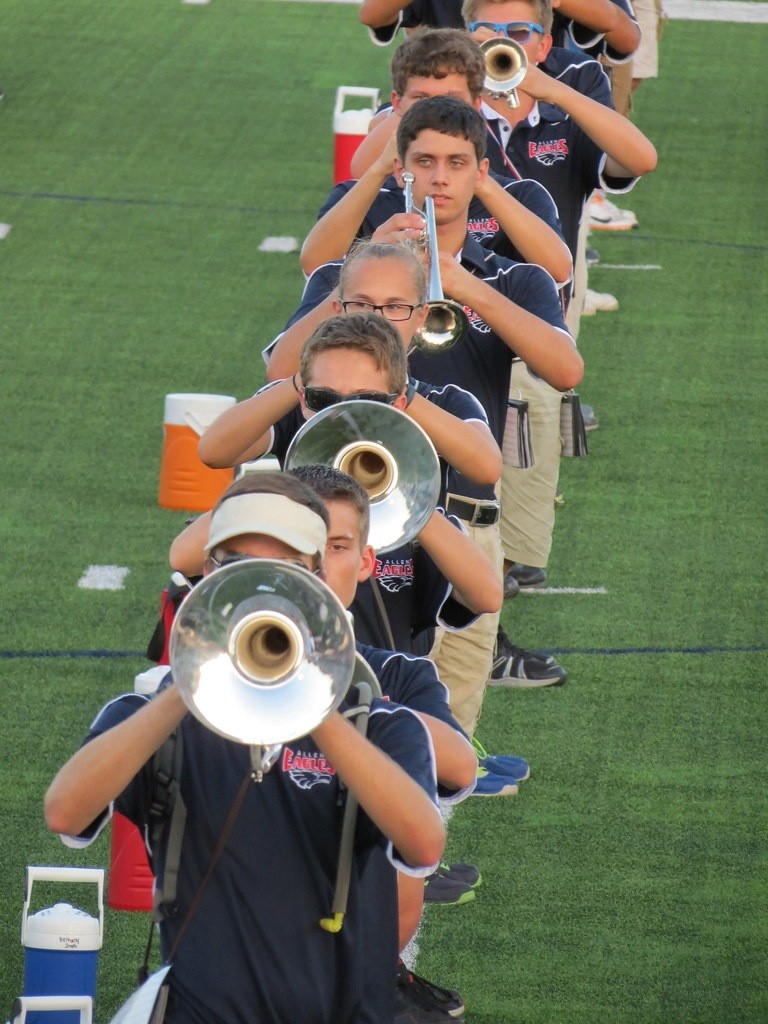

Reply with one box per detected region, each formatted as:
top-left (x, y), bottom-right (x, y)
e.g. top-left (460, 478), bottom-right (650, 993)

top-left (285, 398), bottom-right (442, 556)
top-left (169, 558), bottom-right (358, 778)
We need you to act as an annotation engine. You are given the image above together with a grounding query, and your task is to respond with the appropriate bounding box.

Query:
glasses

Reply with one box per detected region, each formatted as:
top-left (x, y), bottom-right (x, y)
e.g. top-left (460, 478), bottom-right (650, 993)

top-left (302, 386), bottom-right (396, 413)
top-left (208, 554), bottom-right (320, 578)
top-left (336, 299), bottom-right (424, 322)
top-left (470, 22), bottom-right (543, 45)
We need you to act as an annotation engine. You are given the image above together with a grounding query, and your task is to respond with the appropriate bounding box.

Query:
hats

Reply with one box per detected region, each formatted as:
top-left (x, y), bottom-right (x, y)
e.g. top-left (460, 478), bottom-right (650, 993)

top-left (204, 494), bottom-right (328, 561)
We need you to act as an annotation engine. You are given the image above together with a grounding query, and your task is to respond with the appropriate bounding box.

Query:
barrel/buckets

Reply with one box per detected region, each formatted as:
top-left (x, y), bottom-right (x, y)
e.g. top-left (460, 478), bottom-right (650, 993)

top-left (157, 394), bottom-right (237, 513)
top-left (158, 588), bottom-right (189, 666)
top-left (332, 86), bottom-right (382, 187)
top-left (5, 864), bottom-right (103, 1024)
top-left (108, 811), bottom-right (155, 909)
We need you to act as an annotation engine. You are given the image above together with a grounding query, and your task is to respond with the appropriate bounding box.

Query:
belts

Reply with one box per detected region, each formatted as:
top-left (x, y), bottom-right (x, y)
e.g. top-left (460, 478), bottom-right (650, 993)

top-left (444, 497), bottom-right (500, 526)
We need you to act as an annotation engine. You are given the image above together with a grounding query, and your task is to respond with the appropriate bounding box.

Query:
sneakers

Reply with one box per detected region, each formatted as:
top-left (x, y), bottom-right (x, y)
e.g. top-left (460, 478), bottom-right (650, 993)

top-left (422, 860), bottom-right (482, 903)
top-left (581, 288), bottom-right (617, 316)
top-left (585, 193), bottom-right (637, 231)
top-left (470, 739), bottom-right (531, 798)
top-left (391, 955), bottom-right (465, 1017)
top-left (485, 621), bottom-right (566, 690)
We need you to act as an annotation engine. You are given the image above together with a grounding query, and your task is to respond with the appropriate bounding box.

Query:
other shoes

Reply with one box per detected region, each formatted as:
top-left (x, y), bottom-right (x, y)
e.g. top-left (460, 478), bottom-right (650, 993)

top-left (579, 403), bottom-right (599, 433)
top-left (585, 244), bottom-right (599, 265)
top-left (502, 560), bottom-right (546, 600)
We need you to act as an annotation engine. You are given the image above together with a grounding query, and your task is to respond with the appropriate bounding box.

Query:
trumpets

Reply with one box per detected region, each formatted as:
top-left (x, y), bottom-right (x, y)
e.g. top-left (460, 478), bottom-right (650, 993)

top-left (479, 38), bottom-right (529, 109)
top-left (402, 170), bottom-right (470, 355)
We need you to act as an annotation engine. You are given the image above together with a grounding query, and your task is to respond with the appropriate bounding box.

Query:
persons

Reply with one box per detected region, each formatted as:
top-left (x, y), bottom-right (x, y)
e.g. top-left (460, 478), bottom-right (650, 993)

top-left (42, 0), bottom-right (662, 1024)
